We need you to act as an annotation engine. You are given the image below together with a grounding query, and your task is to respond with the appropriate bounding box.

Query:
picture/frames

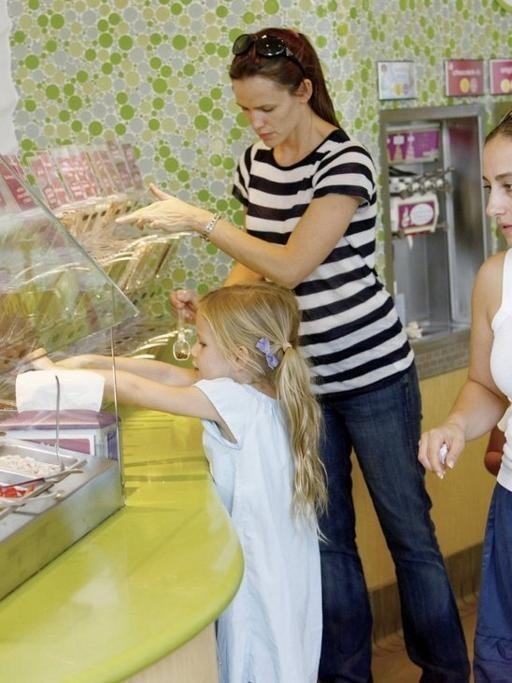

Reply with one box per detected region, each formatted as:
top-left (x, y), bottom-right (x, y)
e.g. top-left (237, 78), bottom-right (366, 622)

top-left (444, 56), bottom-right (483, 97)
top-left (488, 58), bottom-right (511, 97)
top-left (376, 57), bottom-right (418, 101)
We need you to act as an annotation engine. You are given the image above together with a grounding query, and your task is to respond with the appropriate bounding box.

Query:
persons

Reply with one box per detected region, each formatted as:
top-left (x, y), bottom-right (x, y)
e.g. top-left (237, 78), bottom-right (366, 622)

top-left (15, 283), bottom-right (332, 682)
top-left (419, 105), bottom-right (512, 682)
top-left (115, 27), bottom-right (470, 682)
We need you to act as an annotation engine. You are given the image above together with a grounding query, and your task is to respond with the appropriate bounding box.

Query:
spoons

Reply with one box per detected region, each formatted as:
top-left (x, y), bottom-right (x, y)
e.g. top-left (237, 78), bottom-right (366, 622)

top-left (172, 302), bottom-right (191, 360)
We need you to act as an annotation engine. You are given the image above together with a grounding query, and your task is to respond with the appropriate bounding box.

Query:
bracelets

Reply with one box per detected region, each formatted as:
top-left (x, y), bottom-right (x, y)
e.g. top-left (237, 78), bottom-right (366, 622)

top-left (202, 213), bottom-right (218, 241)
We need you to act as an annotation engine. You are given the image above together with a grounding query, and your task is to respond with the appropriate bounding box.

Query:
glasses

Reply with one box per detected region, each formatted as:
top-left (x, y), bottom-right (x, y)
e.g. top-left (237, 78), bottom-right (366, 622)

top-left (233, 34), bottom-right (307, 77)
